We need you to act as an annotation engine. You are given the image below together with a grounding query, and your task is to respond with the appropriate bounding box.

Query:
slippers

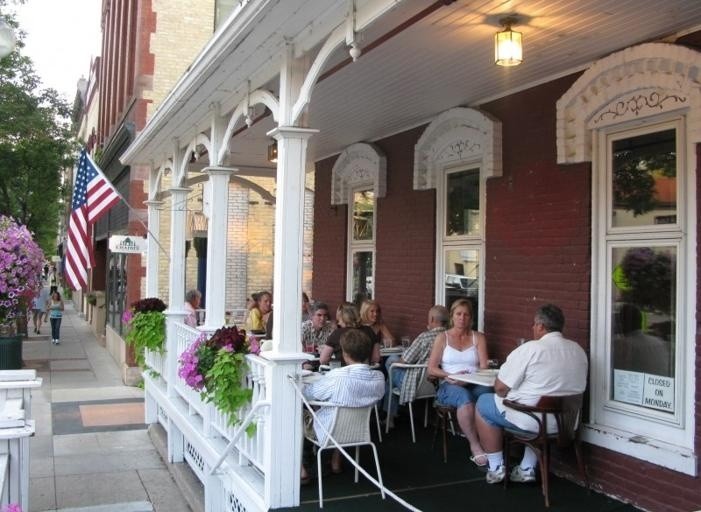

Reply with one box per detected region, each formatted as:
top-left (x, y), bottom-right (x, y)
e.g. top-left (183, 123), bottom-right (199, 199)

top-left (469, 453), bottom-right (488, 467)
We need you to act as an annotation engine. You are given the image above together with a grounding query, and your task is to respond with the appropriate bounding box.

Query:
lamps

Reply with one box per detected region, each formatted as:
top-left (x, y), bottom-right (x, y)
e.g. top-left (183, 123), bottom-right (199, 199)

top-left (495, 17), bottom-right (523, 68)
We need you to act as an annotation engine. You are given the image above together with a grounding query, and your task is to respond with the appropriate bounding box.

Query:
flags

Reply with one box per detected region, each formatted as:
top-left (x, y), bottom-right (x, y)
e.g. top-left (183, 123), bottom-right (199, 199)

top-left (61, 147), bottom-right (124, 293)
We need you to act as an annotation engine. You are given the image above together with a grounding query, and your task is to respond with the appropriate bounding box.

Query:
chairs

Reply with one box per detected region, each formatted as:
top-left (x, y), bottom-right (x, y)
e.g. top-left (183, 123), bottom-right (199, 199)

top-left (319, 364), bottom-right (383, 443)
top-left (501, 392), bottom-right (586, 506)
top-left (302, 399), bottom-right (385, 508)
top-left (425, 375), bottom-right (469, 464)
top-left (385, 364), bottom-right (438, 444)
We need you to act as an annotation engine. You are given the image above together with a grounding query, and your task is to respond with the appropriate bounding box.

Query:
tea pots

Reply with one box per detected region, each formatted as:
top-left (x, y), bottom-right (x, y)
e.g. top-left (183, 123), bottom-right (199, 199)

top-left (259, 338), bottom-right (272, 352)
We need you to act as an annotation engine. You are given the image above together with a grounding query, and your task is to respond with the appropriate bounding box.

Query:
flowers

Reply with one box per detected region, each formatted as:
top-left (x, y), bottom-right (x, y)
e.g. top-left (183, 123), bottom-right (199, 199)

top-left (0, 213), bottom-right (46, 338)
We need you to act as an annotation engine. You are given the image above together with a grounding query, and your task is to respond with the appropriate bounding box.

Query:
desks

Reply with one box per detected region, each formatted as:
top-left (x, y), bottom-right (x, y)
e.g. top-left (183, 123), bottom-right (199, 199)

top-left (380, 348), bottom-right (405, 358)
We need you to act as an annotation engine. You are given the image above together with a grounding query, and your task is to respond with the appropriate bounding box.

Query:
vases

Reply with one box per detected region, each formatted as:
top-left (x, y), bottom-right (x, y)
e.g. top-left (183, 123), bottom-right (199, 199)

top-left (0, 338), bottom-right (23, 370)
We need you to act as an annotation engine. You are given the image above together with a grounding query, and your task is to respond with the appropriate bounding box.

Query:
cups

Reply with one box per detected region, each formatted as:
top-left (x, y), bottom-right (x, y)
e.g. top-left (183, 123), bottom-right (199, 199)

top-left (382, 338), bottom-right (393, 349)
top-left (400, 336), bottom-right (410, 352)
top-left (303, 338), bottom-right (314, 354)
top-left (329, 361), bottom-right (341, 371)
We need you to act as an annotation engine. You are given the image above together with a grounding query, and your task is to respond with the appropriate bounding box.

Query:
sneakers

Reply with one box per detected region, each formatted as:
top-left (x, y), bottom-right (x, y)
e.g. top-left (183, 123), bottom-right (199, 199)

top-left (510, 464), bottom-right (536, 482)
top-left (486, 464), bottom-right (506, 484)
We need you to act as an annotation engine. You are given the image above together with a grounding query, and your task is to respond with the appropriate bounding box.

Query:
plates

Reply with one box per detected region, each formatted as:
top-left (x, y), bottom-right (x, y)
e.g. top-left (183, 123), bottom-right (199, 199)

top-left (301, 368), bottom-right (313, 377)
top-left (471, 367), bottom-right (501, 377)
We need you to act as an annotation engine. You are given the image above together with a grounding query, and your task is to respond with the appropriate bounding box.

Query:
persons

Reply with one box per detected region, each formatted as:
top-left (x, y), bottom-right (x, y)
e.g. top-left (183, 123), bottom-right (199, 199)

top-left (185, 288), bottom-right (202, 329)
top-left (30, 263), bottom-right (64, 345)
top-left (475, 303), bottom-right (589, 484)
top-left (385, 299), bottom-right (488, 466)
top-left (301, 291), bottom-right (393, 483)
top-left (610, 304), bottom-right (669, 376)
top-left (246, 291), bottom-right (273, 340)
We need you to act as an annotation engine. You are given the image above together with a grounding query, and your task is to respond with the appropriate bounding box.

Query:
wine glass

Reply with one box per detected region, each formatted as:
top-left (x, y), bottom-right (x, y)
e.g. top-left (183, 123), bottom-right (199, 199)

top-left (487, 358), bottom-right (500, 381)
